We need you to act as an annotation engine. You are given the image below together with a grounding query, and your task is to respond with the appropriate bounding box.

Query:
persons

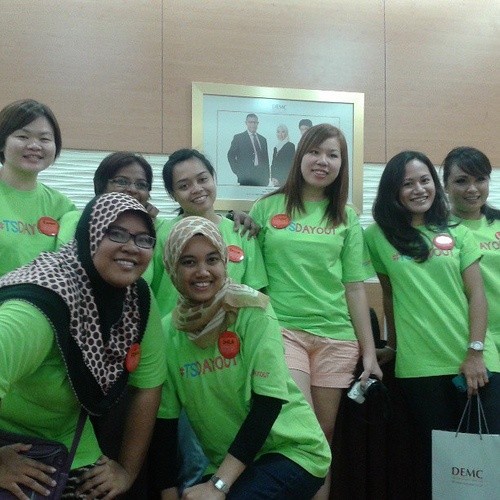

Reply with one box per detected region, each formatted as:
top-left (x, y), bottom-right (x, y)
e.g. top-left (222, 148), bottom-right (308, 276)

top-left (156, 148), bottom-right (269, 320)
top-left (365, 151), bottom-right (488, 500)
top-left (271, 125), bottom-right (295, 187)
top-left (227, 114), bottom-right (270, 187)
top-left (249, 124), bottom-right (383, 500)
top-left (439, 146), bottom-right (500, 434)
top-left (299, 119), bottom-right (312, 135)
top-left (0, 191), bottom-right (168, 500)
top-left (155, 216), bottom-right (332, 500)
top-left (0, 98), bottom-right (78, 278)
top-left (54, 151), bottom-right (261, 298)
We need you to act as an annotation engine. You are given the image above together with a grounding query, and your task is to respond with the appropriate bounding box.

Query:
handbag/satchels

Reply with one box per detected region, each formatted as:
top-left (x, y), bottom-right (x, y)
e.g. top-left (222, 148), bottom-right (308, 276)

top-left (432, 389), bottom-right (500, 500)
top-left (0, 431), bottom-right (70, 500)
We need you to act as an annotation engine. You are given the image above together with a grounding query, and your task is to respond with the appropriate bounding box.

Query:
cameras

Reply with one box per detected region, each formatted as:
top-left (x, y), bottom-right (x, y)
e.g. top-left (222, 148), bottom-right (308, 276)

top-left (347, 377), bottom-right (377, 404)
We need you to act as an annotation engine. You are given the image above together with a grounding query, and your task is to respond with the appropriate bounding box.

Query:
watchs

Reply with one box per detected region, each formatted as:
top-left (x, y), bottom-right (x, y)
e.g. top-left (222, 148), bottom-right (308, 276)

top-left (467, 341), bottom-right (484, 351)
top-left (211, 475), bottom-right (229, 494)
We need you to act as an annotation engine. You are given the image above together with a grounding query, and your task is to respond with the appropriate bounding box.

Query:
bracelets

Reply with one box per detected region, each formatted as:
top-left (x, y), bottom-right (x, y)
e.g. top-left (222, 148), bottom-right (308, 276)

top-left (226, 210), bottom-right (234, 221)
top-left (385, 346), bottom-right (395, 353)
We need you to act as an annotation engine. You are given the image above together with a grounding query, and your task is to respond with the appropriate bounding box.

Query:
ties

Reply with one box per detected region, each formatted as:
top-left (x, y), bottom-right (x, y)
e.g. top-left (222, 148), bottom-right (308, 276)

top-left (253, 133), bottom-right (263, 167)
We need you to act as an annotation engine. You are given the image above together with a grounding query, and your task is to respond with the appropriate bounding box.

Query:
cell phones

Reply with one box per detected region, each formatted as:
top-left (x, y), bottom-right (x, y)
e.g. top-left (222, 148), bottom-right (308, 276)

top-left (452, 367), bottom-right (493, 393)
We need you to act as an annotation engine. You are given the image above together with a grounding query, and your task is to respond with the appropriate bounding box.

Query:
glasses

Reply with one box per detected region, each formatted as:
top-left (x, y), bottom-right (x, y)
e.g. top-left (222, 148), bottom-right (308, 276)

top-left (105, 226), bottom-right (157, 249)
top-left (109, 178), bottom-right (150, 190)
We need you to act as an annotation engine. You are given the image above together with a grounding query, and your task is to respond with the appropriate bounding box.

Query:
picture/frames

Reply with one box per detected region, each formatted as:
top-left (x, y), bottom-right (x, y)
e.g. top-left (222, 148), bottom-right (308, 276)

top-left (191, 80), bottom-right (365, 217)
